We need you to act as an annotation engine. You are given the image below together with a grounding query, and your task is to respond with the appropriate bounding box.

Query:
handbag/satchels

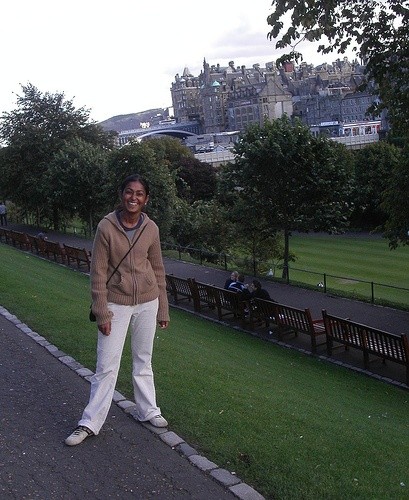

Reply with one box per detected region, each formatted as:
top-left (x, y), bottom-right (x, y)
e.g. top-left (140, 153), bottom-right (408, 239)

top-left (89, 301), bottom-right (96, 321)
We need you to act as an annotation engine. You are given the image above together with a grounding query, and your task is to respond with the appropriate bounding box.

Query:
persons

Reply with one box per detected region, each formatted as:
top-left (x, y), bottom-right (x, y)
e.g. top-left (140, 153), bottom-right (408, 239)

top-left (0, 202), bottom-right (8, 226)
top-left (317, 281), bottom-right (323, 288)
top-left (223, 271), bottom-right (279, 326)
top-left (64, 173), bottom-right (171, 446)
top-left (38, 230), bottom-right (48, 255)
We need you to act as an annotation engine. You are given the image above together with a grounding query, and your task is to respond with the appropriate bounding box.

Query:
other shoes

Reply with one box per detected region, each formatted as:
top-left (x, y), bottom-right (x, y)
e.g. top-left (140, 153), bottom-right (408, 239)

top-left (64, 425), bottom-right (93, 446)
top-left (149, 414), bottom-right (168, 428)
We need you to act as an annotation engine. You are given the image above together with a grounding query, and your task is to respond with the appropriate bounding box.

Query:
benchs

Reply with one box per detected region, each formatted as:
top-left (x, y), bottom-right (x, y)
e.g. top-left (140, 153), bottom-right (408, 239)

top-left (0, 227), bottom-right (409, 388)
